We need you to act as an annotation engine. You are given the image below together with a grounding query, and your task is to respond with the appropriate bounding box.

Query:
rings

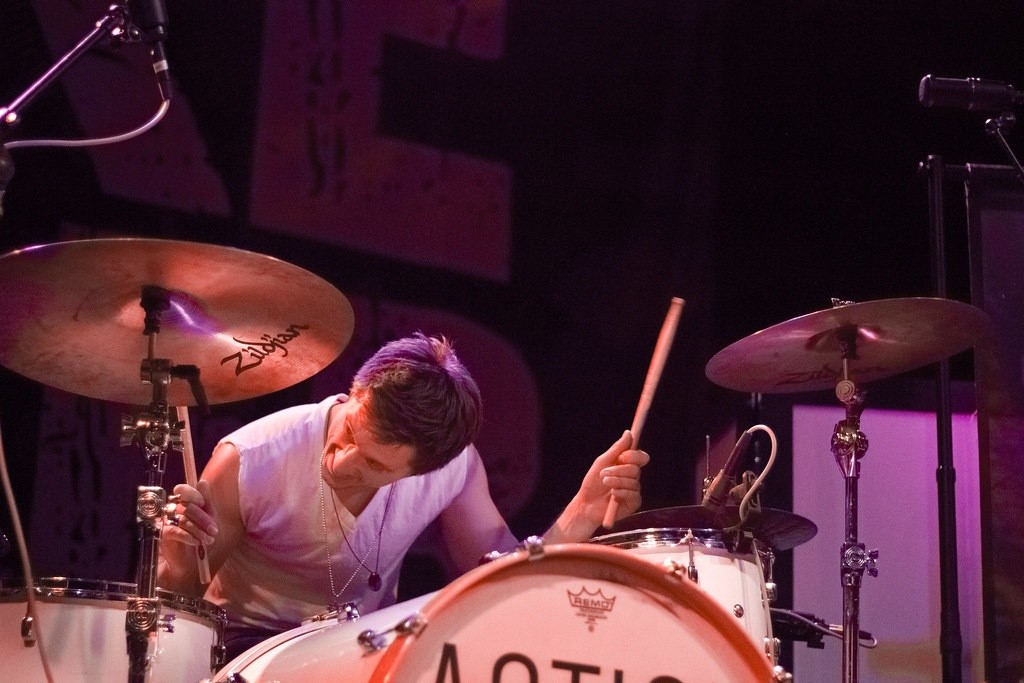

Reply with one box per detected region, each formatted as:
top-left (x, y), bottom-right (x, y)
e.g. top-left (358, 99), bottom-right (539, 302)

top-left (170, 514), bottom-right (181, 525)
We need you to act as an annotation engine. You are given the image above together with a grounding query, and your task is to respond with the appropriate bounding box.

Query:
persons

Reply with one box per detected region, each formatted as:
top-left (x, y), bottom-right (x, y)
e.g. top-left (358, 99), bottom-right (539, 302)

top-left (153, 332), bottom-right (651, 672)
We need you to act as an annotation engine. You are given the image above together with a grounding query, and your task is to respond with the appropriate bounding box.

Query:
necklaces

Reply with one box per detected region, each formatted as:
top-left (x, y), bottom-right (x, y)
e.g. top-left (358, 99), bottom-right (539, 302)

top-left (320, 401), bottom-right (397, 614)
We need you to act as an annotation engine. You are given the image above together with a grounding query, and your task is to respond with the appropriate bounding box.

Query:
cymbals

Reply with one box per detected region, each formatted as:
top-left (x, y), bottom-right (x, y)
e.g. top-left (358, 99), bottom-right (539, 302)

top-left (608, 503), bottom-right (819, 555)
top-left (703, 295), bottom-right (990, 395)
top-left (0, 233), bottom-right (356, 412)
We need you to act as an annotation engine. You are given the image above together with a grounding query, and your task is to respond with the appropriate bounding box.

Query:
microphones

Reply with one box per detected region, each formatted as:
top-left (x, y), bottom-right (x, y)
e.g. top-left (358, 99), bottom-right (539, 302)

top-left (702, 429), bottom-right (752, 513)
top-left (126, 0), bottom-right (173, 99)
top-left (770, 607), bottom-right (871, 644)
top-left (918, 74), bottom-right (1024, 111)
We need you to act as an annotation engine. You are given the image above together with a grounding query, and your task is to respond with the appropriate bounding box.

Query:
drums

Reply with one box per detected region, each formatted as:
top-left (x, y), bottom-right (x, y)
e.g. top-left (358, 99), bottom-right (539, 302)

top-left (201, 535), bottom-right (793, 683)
top-left (572, 526), bottom-right (781, 671)
top-left (0, 575), bottom-right (230, 683)
